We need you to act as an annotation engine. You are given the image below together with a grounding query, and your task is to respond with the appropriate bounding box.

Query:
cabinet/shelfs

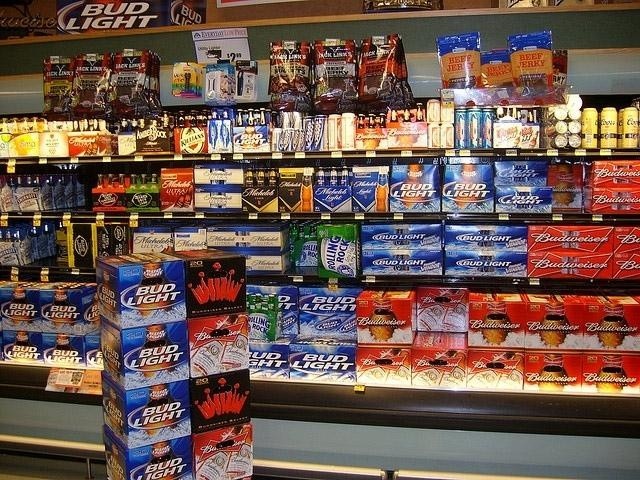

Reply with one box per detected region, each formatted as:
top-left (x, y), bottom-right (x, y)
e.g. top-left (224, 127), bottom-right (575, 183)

top-left (0, 45), bottom-right (640, 480)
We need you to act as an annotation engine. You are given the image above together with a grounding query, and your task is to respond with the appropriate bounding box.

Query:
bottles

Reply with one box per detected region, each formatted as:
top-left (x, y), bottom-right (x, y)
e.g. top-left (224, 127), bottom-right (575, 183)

top-left (375, 165), bottom-right (482, 214)
top-left (194, 424), bottom-right (251, 479)
top-left (1, 97), bottom-right (425, 134)
top-left (555, 163), bottom-right (574, 204)
top-left (316, 167), bottom-right (355, 188)
top-left (288, 220), bottom-right (323, 260)
top-left (300, 172), bottom-right (313, 212)
top-left (95, 173), bottom-right (157, 187)
top-left (101, 268), bottom-right (127, 479)
top-left (9, 290), bottom-right (98, 361)
top-left (248, 292), bottom-right (281, 311)
top-left (174, 182), bottom-right (193, 209)
top-left (12, 173), bottom-right (82, 186)
top-left (0, 224), bottom-right (54, 241)
top-left (190, 314), bottom-right (247, 377)
top-left (135, 264), bottom-right (178, 480)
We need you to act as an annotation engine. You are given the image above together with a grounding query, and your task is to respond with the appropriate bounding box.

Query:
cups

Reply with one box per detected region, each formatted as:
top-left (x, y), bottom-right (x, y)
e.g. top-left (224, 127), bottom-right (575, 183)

top-left (367, 309), bottom-right (628, 395)
top-left (244, 167), bottom-right (277, 186)
